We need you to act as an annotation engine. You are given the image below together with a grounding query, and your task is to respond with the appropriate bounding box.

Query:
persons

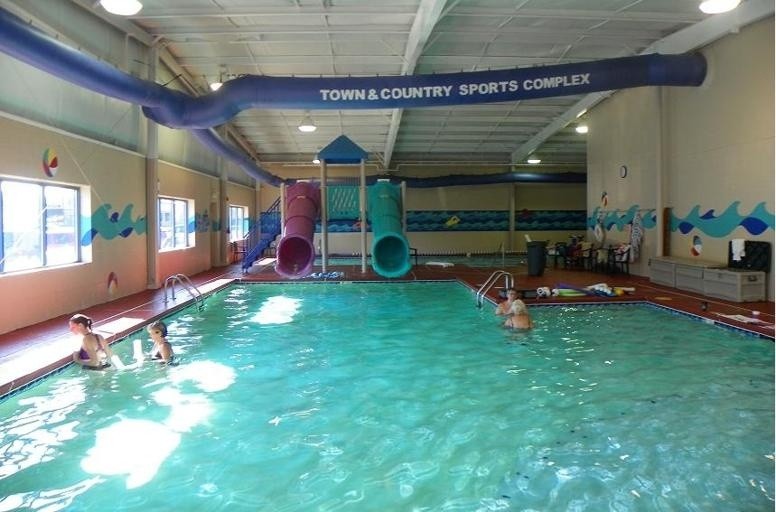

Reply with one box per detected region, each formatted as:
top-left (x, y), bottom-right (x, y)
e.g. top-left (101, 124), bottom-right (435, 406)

top-left (494, 287), bottom-right (517, 315)
top-left (503, 299), bottom-right (533, 330)
top-left (145, 319), bottom-right (176, 363)
top-left (68, 313), bottom-right (113, 371)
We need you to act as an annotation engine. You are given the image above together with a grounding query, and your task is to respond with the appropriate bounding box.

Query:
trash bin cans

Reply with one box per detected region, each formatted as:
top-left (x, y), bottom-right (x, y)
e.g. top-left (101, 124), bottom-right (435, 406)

top-left (527, 241), bottom-right (546, 277)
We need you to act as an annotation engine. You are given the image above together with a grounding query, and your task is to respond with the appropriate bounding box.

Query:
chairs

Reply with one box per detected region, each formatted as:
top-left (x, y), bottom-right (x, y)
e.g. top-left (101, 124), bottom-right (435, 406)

top-left (554, 238), bottom-right (632, 278)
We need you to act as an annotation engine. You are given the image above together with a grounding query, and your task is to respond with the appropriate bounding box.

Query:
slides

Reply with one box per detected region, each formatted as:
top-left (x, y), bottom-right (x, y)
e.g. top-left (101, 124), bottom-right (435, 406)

top-left (367, 183), bottom-right (411, 279)
top-left (274, 181), bottom-right (320, 278)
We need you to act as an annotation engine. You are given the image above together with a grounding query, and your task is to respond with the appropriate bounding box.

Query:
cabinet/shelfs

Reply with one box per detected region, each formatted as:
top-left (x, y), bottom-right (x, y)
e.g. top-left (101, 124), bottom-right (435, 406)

top-left (648, 254), bottom-right (767, 306)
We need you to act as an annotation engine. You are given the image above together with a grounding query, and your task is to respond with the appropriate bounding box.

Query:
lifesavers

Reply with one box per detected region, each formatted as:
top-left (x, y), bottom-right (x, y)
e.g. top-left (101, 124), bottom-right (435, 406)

top-left (594, 225), bottom-right (604, 243)
